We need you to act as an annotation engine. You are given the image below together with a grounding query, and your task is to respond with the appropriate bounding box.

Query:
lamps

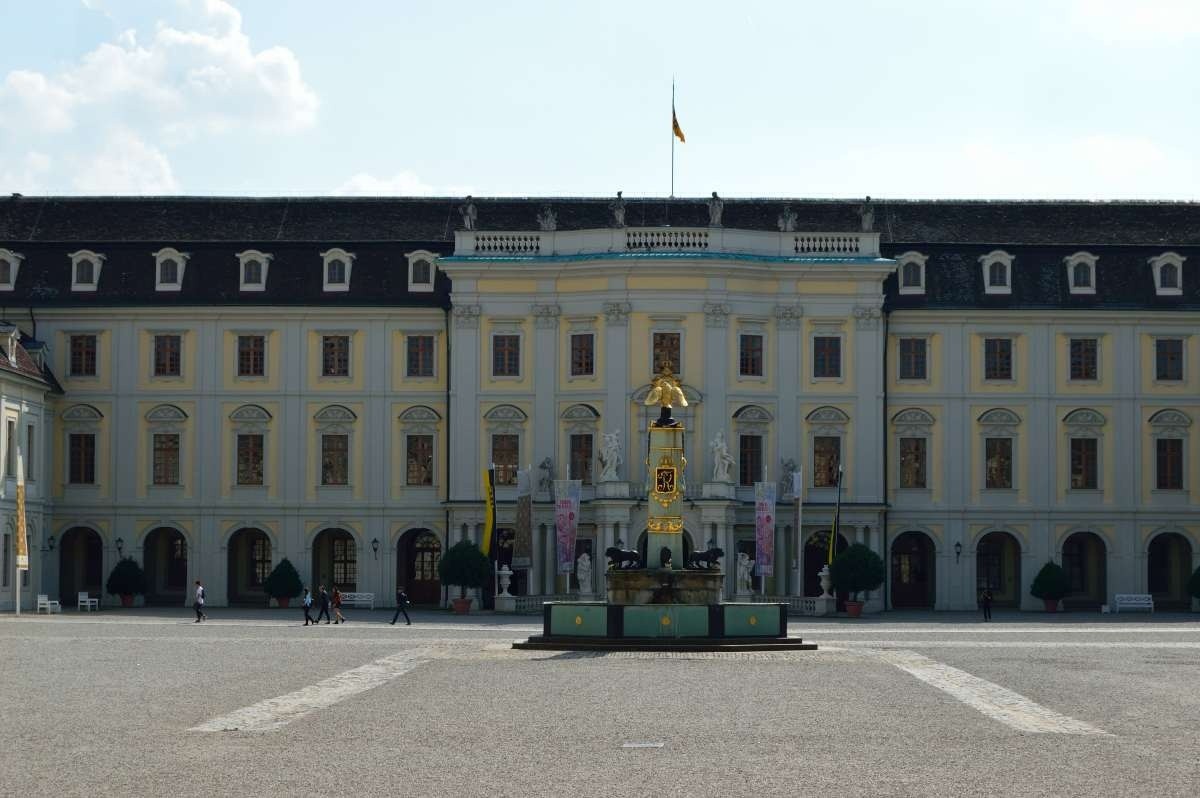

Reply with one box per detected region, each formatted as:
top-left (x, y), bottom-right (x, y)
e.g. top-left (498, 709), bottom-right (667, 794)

top-left (47, 535), bottom-right (56, 550)
top-left (954, 541), bottom-right (962, 563)
top-left (371, 537), bottom-right (380, 560)
top-left (115, 537), bottom-right (125, 555)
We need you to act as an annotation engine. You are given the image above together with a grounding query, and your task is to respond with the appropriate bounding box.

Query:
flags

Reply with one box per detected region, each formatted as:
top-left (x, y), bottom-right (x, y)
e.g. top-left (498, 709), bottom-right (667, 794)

top-left (672, 108), bottom-right (686, 142)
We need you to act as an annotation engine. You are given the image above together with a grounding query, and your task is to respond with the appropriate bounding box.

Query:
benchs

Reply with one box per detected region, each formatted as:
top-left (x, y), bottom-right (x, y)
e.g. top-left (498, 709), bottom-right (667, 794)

top-left (1115, 594), bottom-right (1154, 615)
top-left (341, 593), bottom-right (376, 610)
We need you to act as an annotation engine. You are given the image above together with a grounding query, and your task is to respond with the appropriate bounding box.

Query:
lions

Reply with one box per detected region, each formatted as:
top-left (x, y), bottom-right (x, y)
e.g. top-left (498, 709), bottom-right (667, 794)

top-left (690, 548), bottom-right (724, 568)
top-left (605, 547), bottom-right (640, 569)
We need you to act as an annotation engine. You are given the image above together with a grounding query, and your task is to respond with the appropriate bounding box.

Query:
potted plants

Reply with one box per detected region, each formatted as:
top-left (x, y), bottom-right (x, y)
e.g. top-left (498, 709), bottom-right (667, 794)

top-left (1028, 560), bottom-right (1068, 613)
top-left (437, 535), bottom-right (496, 617)
top-left (264, 557), bottom-right (303, 606)
top-left (105, 556), bottom-right (149, 606)
top-left (828, 542), bottom-right (886, 618)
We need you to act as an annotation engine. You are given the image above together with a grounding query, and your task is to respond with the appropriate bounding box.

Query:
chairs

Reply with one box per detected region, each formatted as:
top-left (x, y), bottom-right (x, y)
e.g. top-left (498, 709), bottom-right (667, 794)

top-left (77, 591), bottom-right (99, 613)
top-left (38, 594), bottom-right (59, 615)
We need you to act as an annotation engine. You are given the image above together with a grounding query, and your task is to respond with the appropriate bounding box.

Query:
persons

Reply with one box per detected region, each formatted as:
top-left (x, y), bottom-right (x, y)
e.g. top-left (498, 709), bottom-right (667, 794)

top-left (539, 456), bottom-right (553, 492)
top-left (303, 588), bottom-right (315, 626)
top-left (193, 581), bottom-right (206, 623)
top-left (390, 587), bottom-right (411, 624)
top-left (332, 586), bottom-right (346, 624)
top-left (314, 586), bottom-right (330, 624)
top-left (710, 432), bottom-right (736, 481)
top-left (599, 429), bottom-right (623, 481)
top-left (457, 192), bottom-right (875, 234)
top-left (980, 588), bottom-right (992, 623)
top-left (780, 457), bottom-right (796, 493)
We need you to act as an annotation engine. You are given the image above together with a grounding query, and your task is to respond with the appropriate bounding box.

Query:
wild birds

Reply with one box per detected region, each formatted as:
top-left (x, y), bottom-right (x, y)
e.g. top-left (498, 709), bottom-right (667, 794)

top-left (644, 381), bottom-right (689, 407)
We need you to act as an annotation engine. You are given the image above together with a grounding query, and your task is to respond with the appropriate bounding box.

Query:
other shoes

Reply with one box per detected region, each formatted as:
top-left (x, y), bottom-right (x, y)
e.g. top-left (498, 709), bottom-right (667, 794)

top-left (312, 620), bottom-right (314, 624)
top-left (389, 622), bottom-right (394, 624)
top-left (315, 620), bottom-right (318, 623)
top-left (407, 622), bottom-right (411, 625)
top-left (302, 623), bottom-right (308, 626)
top-left (203, 615), bottom-right (206, 620)
top-left (325, 621), bottom-right (330, 624)
top-left (195, 620), bottom-right (199, 623)
top-left (342, 617), bottom-right (345, 623)
top-left (332, 620), bottom-right (338, 624)
